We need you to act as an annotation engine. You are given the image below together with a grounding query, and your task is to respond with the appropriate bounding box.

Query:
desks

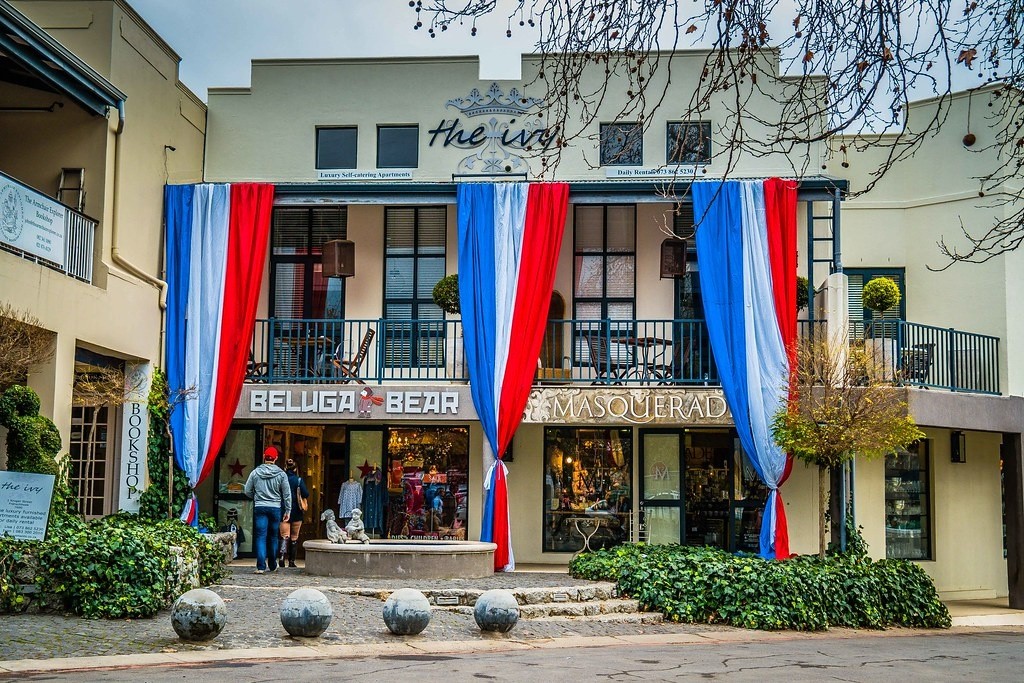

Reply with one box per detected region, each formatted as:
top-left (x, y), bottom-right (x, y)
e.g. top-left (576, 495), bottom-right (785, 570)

top-left (565, 518), bottom-right (609, 561)
top-left (545, 511), bottom-right (630, 550)
top-left (279, 336), bottom-right (336, 384)
top-left (611, 337), bottom-right (673, 387)
top-left (900, 348), bottom-right (927, 355)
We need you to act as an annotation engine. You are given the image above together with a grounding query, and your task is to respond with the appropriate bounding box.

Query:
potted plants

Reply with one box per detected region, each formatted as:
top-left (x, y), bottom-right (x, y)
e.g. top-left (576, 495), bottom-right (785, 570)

top-left (861, 276), bottom-right (902, 381)
top-left (432, 274), bottom-right (471, 385)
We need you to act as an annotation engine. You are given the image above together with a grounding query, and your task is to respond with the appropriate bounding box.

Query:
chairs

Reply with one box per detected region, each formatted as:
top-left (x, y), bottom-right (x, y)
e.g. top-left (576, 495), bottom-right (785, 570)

top-left (648, 336), bottom-right (696, 386)
top-left (330, 328), bottom-right (375, 385)
top-left (906, 343), bottom-right (936, 390)
top-left (538, 330), bottom-right (573, 386)
top-left (242, 348), bottom-right (279, 383)
top-left (585, 335), bottom-right (632, 386)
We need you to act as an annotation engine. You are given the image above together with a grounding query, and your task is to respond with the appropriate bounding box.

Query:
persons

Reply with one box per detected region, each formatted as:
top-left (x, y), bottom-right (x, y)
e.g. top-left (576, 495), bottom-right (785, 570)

top-left (277, 458), bottom-right (309, 568)
top-left (442, 484), bottom-right (459, 526)
top-left (431, 487), bottom-right (445, 526)
top-left (243, 447), bottom-right (292, 574)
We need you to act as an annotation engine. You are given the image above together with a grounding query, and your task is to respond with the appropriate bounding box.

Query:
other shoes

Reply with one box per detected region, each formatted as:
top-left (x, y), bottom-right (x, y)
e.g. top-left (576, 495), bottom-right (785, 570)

top-left (252, 569), bottom-right (265, 573)
top-left (271, 562), bottom-right (279, 572)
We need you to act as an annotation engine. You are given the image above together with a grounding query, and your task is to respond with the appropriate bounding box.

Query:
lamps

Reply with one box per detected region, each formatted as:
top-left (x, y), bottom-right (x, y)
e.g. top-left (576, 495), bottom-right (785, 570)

top-left (660, 238), bottom-right (688, 279)
top-left (320, 205), bottom-right (356, 278)
top-left (951, 430), bottom-right (966, 463)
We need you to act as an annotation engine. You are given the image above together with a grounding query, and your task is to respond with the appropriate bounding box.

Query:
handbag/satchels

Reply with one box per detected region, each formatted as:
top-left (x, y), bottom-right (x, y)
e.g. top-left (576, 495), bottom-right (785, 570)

top-left (296, 477), bottom-right (308, 511)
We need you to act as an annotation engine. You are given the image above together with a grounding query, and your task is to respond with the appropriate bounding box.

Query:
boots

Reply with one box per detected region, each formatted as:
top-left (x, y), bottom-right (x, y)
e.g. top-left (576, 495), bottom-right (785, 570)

top-left (279, 539), bottom-right (288, 567)
top-left (289, 539), bottom-right (297, 567)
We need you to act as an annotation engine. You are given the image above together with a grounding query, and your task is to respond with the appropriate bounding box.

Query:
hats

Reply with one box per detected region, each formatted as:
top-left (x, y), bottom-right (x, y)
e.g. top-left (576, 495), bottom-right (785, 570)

top-left (264, 447), bottom-right (278, 458)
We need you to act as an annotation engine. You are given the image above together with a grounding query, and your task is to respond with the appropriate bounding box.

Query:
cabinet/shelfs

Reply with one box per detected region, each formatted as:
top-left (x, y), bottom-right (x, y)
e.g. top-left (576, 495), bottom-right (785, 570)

top-left (263, 425), bottom-right (324, 559)
top-left (884, 467), bottom-right (929, 559)
